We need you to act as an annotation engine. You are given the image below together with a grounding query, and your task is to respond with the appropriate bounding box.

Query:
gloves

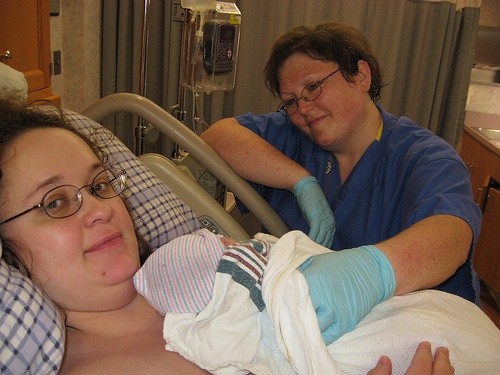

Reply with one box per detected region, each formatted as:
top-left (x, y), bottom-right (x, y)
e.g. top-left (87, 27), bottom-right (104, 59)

top-left (296, 244), bottom-right (396, 347)
top-left (292, 178), bottom-right (336, 249)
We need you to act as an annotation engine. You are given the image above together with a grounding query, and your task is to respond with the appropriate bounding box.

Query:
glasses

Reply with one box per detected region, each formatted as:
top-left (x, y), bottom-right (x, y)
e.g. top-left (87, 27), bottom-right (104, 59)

top-left (0, 168), bottom-right (127, 227)
top-left (275, 66), bottom-right (342, 116)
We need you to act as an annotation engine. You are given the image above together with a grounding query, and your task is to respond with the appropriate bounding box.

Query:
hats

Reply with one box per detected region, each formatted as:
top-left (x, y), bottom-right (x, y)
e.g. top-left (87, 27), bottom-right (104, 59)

top-left (133, 227), bottom-right (226, 317)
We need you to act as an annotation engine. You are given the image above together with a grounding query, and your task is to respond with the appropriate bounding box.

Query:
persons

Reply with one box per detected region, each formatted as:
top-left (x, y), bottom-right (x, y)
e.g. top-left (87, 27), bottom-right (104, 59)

top-left (0, 96), bottom-right (455, 375)
top-left (133, 227), bottom-right (500, 375)
top-left (199, 21), bottom-right (484, 346)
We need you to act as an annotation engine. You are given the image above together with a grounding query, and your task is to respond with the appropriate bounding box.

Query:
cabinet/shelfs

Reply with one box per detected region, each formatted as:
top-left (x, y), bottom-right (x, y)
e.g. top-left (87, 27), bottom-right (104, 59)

top-left (461, 125), bottom-right (500, 306)
top-left (0, 0), bottom-right (49, 100)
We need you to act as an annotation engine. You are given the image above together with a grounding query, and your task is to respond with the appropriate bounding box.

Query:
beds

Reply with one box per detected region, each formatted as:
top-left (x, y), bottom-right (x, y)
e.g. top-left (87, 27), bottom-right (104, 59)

top-left (0, 92), bottom-right (500, 375)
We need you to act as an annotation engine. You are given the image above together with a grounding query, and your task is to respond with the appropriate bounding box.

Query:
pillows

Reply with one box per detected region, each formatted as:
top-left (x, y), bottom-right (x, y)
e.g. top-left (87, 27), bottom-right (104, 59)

top-left (0, 105), bottom-right (199, 375)
